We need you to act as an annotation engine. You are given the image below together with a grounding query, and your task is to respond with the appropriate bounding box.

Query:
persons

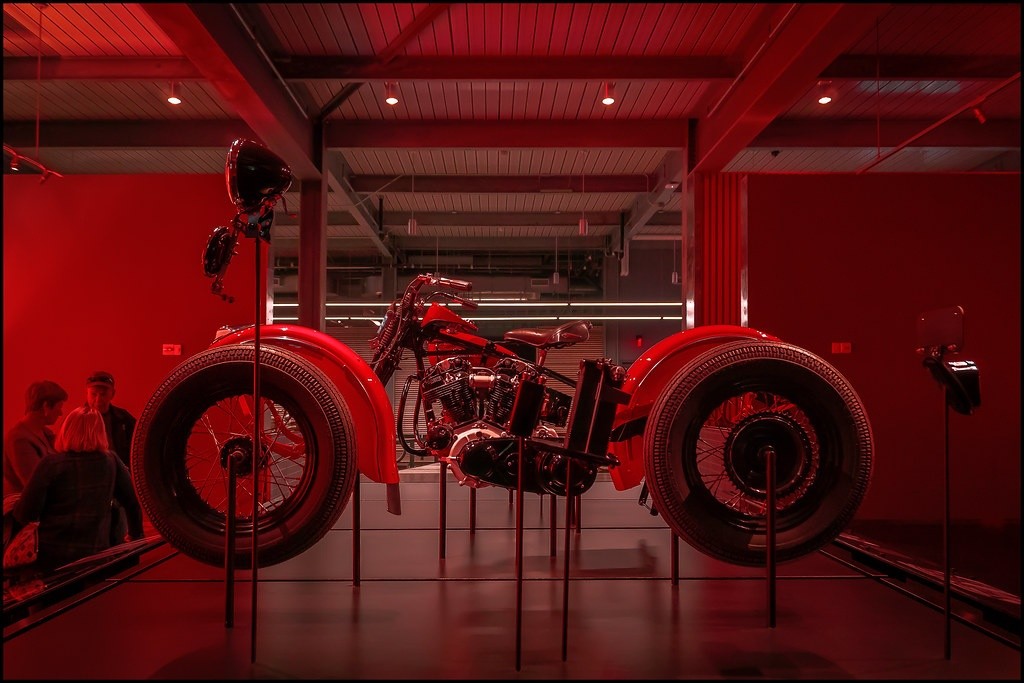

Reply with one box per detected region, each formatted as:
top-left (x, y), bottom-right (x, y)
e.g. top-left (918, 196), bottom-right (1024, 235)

top-left (2, 407), bottom-right (144, 563)
top-left (84, 371), bottom-right (136, 474)
top-left (0, 380), bottom-right (70, 501)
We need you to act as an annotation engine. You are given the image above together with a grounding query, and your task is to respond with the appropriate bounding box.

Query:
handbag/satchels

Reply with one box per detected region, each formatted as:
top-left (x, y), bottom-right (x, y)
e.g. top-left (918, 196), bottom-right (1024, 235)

top-left (2, 521), bottom-right (41, 568)
top-left (110, 451), bottom-right (131, 544)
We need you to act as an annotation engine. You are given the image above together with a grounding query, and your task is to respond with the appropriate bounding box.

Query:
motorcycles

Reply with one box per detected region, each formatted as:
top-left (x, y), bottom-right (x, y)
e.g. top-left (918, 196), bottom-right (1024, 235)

top-left (127, 271), bottom-right (876, 574)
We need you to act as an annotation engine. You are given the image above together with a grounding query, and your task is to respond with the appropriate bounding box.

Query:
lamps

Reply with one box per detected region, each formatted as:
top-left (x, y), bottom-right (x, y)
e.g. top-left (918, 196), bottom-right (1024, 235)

top-left (975, 105), bottom-right (988, 123)
top-left (601, 81), bottom-right (616, 105)
top-left (817, 81), bottom-right (832, 104)
top-left (168, 81), bottom-right (182, 104)
top-left (385, 81), bottom-right (398, 105)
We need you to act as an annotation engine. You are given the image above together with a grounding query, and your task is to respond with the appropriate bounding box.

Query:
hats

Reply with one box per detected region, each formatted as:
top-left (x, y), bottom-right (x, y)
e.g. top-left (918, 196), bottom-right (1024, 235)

top-left (86, 371), bottom-right (114, 388)
